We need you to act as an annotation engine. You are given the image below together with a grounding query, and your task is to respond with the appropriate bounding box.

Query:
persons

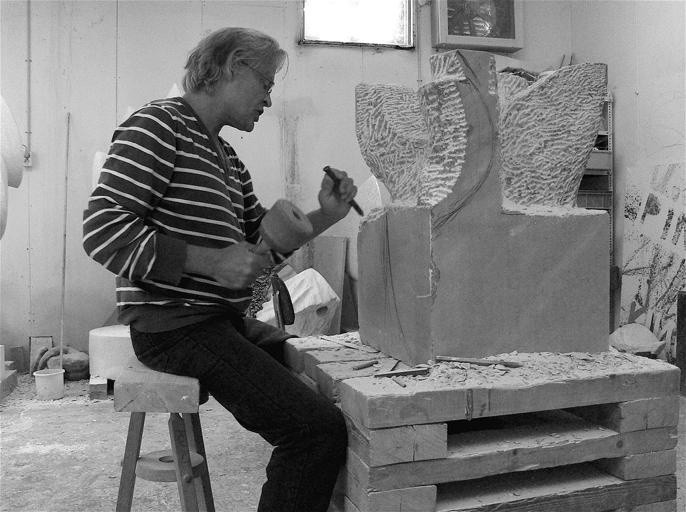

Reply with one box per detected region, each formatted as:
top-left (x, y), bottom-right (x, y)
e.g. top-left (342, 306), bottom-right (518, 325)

top-left (80, 25), bottom-right (359, 511)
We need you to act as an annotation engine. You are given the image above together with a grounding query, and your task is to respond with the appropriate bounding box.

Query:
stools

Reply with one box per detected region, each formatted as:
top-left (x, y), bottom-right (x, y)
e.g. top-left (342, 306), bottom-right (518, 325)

top-left (114, 350), bottom-right (215, 512)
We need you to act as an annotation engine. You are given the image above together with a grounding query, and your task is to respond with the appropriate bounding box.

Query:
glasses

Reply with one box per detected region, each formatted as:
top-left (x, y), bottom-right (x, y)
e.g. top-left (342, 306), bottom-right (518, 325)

top-left (234, 52), bottom-right (275, 95)
